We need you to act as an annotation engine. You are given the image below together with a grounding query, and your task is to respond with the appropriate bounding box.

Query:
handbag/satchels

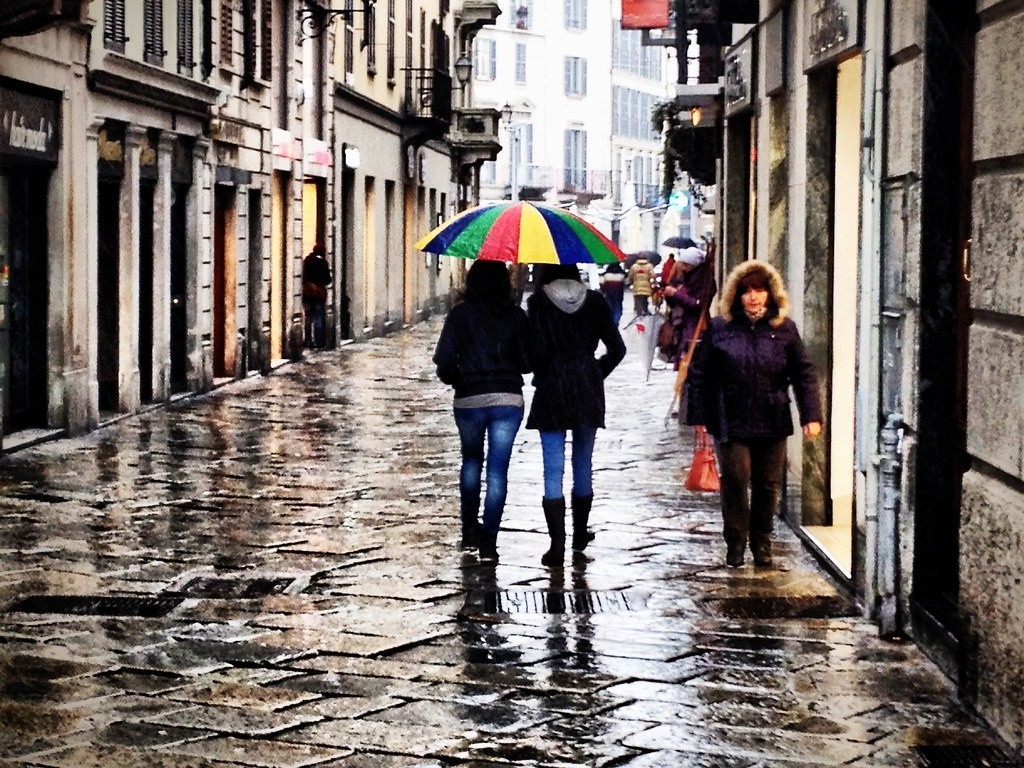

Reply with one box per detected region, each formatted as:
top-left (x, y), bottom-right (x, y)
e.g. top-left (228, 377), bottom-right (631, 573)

top-left (658, 318), bottom-right (676, 349)
top-left (684, 428), bottom-right (721, 492)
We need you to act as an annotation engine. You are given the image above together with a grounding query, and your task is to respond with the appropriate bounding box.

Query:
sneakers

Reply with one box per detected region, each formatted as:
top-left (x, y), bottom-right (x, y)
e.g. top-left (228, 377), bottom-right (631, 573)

top-left (726, 541), bottom-right (746, 566)
top-left (749, 540), bottom-right (773, 568)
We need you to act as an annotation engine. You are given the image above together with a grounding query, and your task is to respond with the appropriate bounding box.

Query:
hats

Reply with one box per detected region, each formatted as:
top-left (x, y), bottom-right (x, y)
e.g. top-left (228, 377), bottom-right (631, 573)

top-left (679, 246), bottom-right (707, 266)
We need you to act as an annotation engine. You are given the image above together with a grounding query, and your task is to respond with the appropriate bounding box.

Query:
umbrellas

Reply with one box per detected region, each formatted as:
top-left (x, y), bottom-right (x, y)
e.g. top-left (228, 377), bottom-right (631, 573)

top-left (664, 237), bottom-right (698, 249)
top-left (414, 187), bottom-right (630, 264)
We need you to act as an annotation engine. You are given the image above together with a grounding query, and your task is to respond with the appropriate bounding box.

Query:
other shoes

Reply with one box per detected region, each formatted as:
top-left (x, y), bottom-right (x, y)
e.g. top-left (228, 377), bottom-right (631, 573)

top-left (458, 535), bottom-right (500, 563)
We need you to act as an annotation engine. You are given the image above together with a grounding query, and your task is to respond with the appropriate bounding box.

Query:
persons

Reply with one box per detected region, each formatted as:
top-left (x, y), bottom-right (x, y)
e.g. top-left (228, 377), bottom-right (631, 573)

top-left (686, 259), bottom-right (824, 567)
top-left (526, 264), bottom-right (627, 560)
top-left (303, 245), bottom-right (332, 348)
top-left (629, 253), bottom-right (656, 317)
top-left (432, 260), bottom-right (549, 559)
top-left (652, 254), bottom-right (716, 363)
top-left (603, 264), bottom-right (625, 330)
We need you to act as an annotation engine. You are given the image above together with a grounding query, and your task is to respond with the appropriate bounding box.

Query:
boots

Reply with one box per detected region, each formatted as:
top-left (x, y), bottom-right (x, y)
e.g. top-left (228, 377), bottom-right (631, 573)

top-left (540, 496), bottom-right (566, 568)
top-left (569, 487), bottom-right (596, 552)
